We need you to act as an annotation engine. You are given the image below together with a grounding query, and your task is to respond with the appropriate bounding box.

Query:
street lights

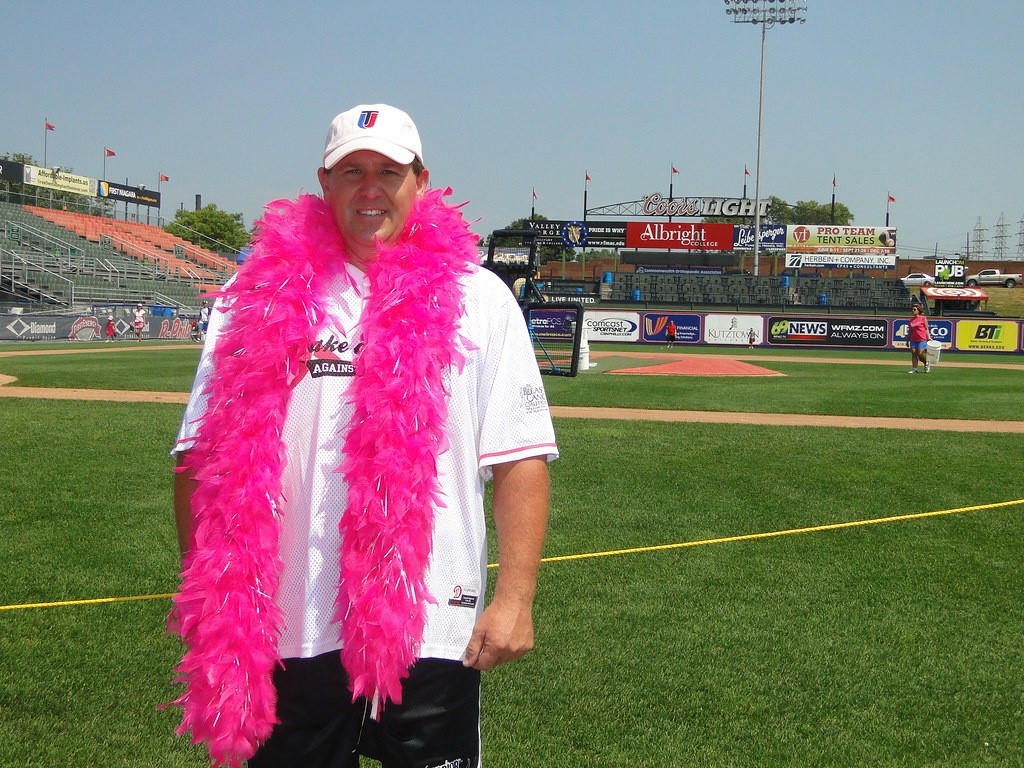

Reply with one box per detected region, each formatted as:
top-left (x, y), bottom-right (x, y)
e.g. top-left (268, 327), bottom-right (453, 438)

top-left (724, 0), bottom-right (808, 276)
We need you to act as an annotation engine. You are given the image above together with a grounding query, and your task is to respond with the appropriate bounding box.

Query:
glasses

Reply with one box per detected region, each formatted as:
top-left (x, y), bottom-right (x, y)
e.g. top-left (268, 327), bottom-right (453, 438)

top-left (911, 309), bottom-right (918, 311)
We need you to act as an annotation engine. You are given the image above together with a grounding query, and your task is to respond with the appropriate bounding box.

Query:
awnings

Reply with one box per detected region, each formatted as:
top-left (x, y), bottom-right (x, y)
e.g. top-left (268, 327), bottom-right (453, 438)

top-left (920, 285), bottom-right (988, 299)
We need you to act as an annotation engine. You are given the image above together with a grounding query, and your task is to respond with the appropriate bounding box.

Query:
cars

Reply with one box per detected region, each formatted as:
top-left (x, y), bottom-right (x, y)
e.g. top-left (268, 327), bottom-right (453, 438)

top-left (900, 272), bottom-right (935, 286)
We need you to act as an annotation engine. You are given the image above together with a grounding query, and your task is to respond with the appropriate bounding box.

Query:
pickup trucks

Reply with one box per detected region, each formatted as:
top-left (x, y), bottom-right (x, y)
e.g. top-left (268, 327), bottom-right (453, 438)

top-left (964, 268), bottom-right (1023, 288)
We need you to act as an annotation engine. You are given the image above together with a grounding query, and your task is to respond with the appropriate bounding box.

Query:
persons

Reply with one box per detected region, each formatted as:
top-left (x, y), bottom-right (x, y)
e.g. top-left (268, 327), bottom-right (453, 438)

top-left (190, 301), bottom-right (211, 341)
top-left (105, 315), bottom-right (116, 343)
top-left (531, 281), bottom-right (545, 303)
top-left (742, 267), bottom-right (752, 277)
top-left (906, 303), bottom-right (933, 374)
top-left (168, 103), bottom-right (558, 768)
top-left (666, 320), bottom-right (676, 348)
top-left (747, 327), bottom-right (758, 349)
top-left (133, 303), bottom-right (146, 343)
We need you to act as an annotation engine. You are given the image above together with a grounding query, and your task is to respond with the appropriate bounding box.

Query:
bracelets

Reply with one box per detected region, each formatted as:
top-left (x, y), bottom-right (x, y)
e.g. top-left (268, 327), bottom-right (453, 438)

top-left (928, 338), bottom-right (931, 340)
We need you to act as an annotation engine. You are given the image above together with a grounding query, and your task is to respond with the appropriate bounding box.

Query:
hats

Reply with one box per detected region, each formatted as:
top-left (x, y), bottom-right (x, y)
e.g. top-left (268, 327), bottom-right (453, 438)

top-left (107, 316), bottom-right (114, 321)
top-left (203, 300), bottom-right (208, 304)
top-left (137, 303), bottom-right (143, 307)
top-left (322, 104), bottom-right (424, 171)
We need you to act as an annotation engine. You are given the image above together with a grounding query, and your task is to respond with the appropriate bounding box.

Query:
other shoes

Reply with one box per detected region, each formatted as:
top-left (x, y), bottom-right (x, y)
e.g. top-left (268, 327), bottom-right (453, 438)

top-left (908, 368), bottom-right (918, 374)
top-left (924, 361), bottom-right (930, 374)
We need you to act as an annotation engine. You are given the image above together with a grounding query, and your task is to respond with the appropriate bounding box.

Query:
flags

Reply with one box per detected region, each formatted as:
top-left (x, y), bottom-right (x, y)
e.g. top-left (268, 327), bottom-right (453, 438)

top-left (161, 175), bottom-right (169, 181)
top-left (745, 169), bottom-right (750, 177)
top-left (533, 191), bottom-right (537, 199)
top-left (672, 167), bottom-right (679, 174)
top-left (46, 123), bottom-right (55, 131)
top-left (833, 177), bottom-right (835, 186)
top-left (586, 175), bottom-right (590, 180)
top-left (888, 196), bottom-right (895, 202)
top-left (107, 149), bottom-right (116, 157)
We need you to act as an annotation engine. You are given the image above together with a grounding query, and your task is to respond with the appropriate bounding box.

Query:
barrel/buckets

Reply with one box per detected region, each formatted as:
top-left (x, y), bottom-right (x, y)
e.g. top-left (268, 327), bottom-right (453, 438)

top-left (536, 282), bottom-right (544, 291)
top-left (152, 305), bottom-right (173, 316)
top-left (926, 340), bottom-right (941, 364)
top-left (604, 272), bottom-right (613, 283)
top-left (632, 290), bottom-right (641, 302)
top-left (519, 284), bottom-right (525, 299)
top-left (818, 294), bottom-right (827, 305)
top-left (576, 288), bottom-right (583, 294)
top-left (783, 276), bottom-right (790, 288)
